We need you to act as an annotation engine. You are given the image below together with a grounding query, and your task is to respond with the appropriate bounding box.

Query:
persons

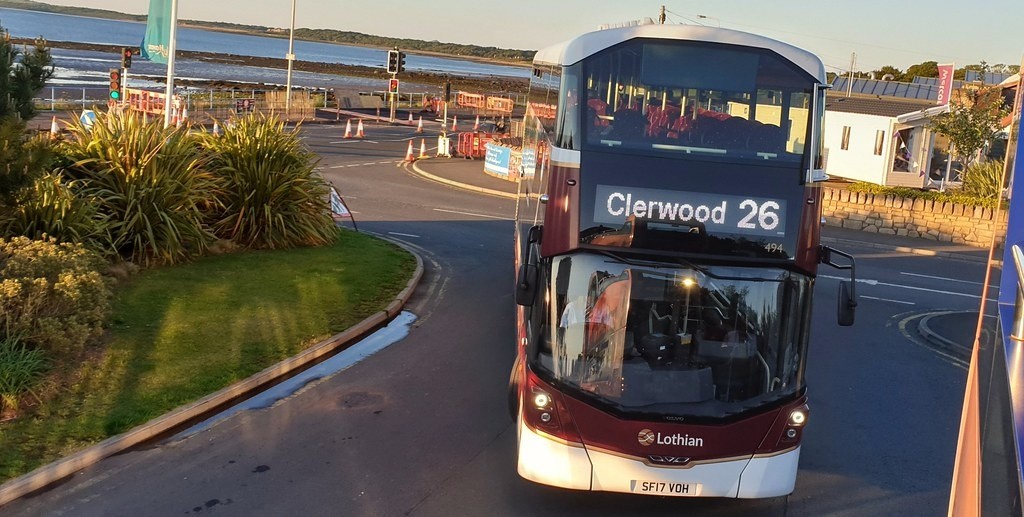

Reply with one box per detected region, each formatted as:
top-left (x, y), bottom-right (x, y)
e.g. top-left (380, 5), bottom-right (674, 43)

top-left (560, 295), bottom-right (613, 328)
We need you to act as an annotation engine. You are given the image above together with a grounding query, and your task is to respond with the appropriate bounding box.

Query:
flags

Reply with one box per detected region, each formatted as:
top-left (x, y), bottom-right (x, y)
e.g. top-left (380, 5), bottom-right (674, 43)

top-left (140, 0), bottom-right (172, 65)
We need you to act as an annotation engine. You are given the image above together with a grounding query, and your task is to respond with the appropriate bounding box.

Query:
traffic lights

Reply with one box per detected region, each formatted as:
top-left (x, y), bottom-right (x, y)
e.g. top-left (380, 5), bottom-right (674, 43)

top-left (389, 79), bottom-right (399, 95)
top-left (123, 48), bottom-right (132, 69)
top-left (387, 49), bottom-right (399, 74)
top-left (109, 68), bottom-right (120, 104)
top-left (399, 51), bottom-right (406, 73)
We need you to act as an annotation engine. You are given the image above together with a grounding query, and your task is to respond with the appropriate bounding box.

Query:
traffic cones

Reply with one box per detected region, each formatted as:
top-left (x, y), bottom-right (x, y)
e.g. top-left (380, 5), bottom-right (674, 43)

top-left (417, 138), bottom-right (429, 160)
top-left (211, 120), bottom-right (222, 138)
top-left (406, 111), bottom-right (414, 127)
top-left (450, 115), bottom-right (458, 133)
top-left (416, 115), bottom-right (424, 134)
top-left (471, 114), bottom-right (481, 132)
top-left (49, 115), bottom-right (62, 138)
top-left (342, 118), bottom-right (353, 139)
top-left (403, 139), bottom-right (417, 162)
top-left (170, 104), bottom-right (189, 131)
top-left (353, 119), bottom-right (366, 140)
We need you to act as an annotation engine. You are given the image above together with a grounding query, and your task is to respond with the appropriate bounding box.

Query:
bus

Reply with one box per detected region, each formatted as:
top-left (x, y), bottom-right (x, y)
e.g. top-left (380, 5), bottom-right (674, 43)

top-left (506, 6), bottom-right (860, 499)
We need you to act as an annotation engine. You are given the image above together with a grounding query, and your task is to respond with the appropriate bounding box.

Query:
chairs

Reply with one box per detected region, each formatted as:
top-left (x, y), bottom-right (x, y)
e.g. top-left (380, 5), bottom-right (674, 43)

top-left (587, 94), bottom-right (786, 150)
